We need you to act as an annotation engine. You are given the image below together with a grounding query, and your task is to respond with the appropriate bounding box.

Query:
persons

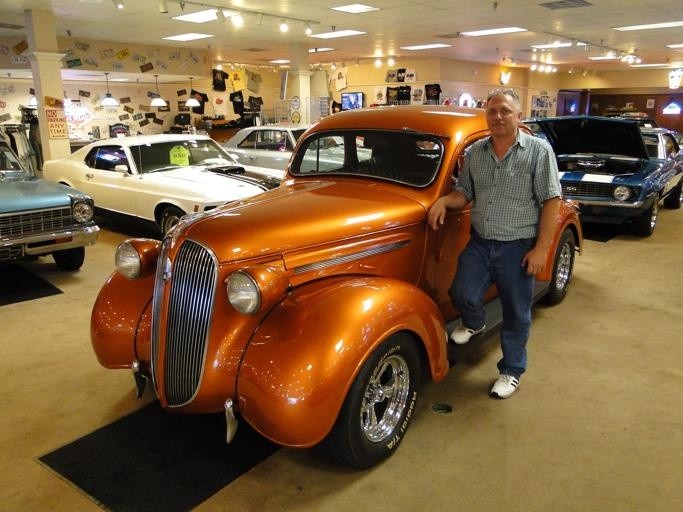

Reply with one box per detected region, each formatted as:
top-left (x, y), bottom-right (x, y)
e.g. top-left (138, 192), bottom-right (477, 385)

top-left (427, 88), bottom-right (563, 399)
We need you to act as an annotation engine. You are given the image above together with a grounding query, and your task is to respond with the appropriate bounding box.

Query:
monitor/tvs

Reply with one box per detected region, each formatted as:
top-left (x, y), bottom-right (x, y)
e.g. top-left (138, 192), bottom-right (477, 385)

top-left (341, 92), bottom-right (363, 111)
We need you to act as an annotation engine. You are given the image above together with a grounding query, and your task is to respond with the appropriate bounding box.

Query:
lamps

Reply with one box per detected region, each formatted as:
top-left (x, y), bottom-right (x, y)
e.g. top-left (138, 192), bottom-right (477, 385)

top-left (151, 75), bottom-right (167, 107)
top-left (185, 77), bottom-right (200, 107)
top-left (310, 59), bottom-right (359, 71)
top-left (157, 0), bottom-right (320, 34)
top-left (101, 73), bottom-right (119, 106)
top-left (500, 31), bottom-right (641, 84)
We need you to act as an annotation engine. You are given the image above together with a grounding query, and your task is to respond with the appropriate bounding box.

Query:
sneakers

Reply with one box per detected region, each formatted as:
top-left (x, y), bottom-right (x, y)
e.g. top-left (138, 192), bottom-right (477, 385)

top-left (449, 323), bottom-right (486, 346)
top-left (490, 374), bottom-right (521, 399)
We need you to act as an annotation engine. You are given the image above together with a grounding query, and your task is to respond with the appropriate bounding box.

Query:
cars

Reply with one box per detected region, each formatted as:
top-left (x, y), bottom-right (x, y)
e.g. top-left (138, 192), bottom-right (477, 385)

top-left (184, 122), bottom-right (372, 177)
top-left (40, 130), bottom-right (286, 248)
top-left (85, 100), bottom-right (584, 481)
top-left (611, 113), bottom-right (661, 130)
top-left (523, 116), bottom-right (549, 142)
top-left (1, 120), bottom-right (102, 275)
top-left (529, 113), bottom-right (681, 238)
